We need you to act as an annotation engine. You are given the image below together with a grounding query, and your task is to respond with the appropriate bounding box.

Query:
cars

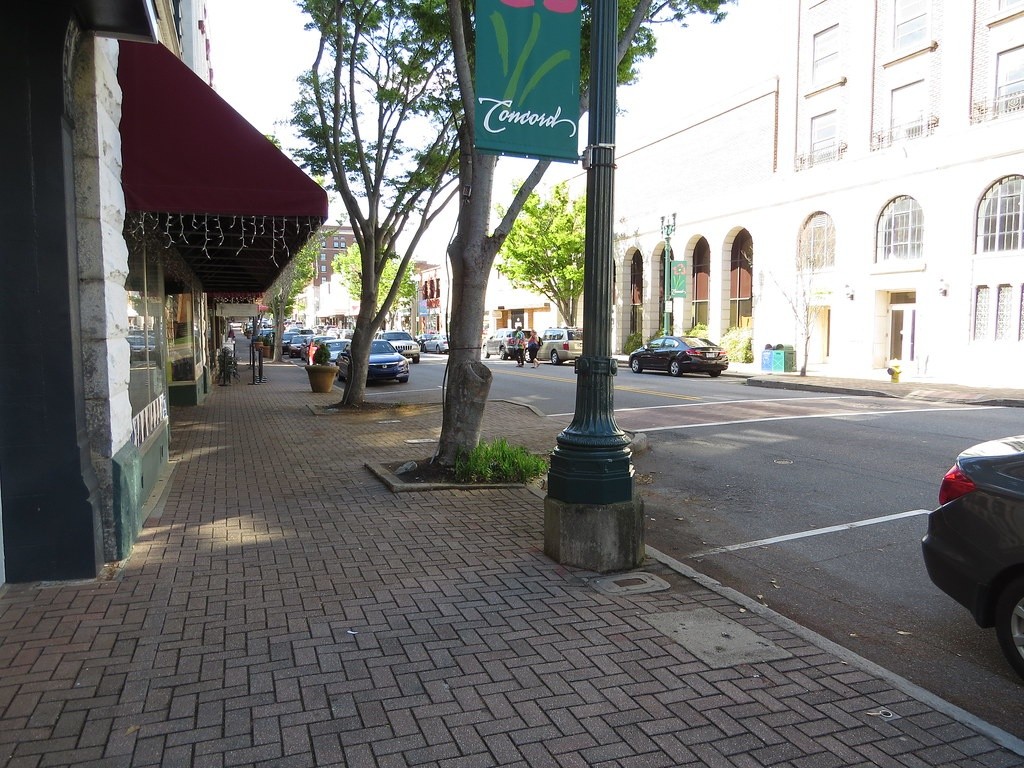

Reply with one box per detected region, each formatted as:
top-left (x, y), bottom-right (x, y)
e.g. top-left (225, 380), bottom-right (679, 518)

top-left (921, 434), bottom-right (1023, 681)
top-left (126, 326), bottom-right (164, 364)
top-left (334, 339), bottom-right (410, 387)
top-left (243, 316), bottom-right (356, 364)
top-left (423, 333), bottom-right (451, 354)
top-left (413, 334), bottom-right (440, 352)
top-left (628, 335), bottom-right (729, 377)
top-left (321, 338), bottom-right (352, 362)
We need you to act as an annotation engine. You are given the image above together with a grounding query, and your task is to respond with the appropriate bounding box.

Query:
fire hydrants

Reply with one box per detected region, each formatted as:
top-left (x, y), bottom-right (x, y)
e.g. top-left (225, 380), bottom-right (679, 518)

top-left (887, 357), bottom-right (903, 385)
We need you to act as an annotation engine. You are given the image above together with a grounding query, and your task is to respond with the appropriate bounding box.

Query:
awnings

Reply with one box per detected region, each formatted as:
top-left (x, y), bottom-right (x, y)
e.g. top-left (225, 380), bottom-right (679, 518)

top-left (118, 36), bottom-right (329, 300)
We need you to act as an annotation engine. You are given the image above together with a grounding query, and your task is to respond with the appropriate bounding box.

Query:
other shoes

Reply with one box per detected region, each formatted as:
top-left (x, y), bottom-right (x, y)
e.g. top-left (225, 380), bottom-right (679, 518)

top-left (516, 364), bottom-right (520, 367)
top-left (530, 366), bottom-right (536, 368)
top-left (519, 364), bottom-right (524, 367)
top-left (536, 362), bottom-right (540, 367)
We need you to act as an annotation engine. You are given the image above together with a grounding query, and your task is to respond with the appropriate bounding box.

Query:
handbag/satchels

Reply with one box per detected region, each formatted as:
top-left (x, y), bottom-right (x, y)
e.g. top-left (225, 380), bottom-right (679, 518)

top-left (539, 337), bottom-right (543, 347)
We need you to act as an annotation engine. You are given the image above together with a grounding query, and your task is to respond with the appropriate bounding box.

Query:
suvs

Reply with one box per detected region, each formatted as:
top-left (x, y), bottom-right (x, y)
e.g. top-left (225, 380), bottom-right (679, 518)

top-left (521, 327), bottom-right (583, 366)
top-left (481, 327), bottom-right (532, 360)
top-left (374, 329), bottom-right (422, 364)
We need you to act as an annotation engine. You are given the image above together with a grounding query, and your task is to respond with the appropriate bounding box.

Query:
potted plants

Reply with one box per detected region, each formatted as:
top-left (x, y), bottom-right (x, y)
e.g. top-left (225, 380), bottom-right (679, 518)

top-left (244, 328), bottom-right (253, 339)
top-left (254, 336), bottom-right (265, 350)
top-left (262, 339), bottom-right (272, 358)
top-left (304, 344), bottom-right (340, 393)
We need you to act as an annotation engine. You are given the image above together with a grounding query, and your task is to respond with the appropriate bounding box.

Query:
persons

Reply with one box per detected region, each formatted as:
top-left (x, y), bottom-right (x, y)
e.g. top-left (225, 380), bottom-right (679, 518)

top-left (514, 326), bottom-right (525, 367)
top-left (528, 330), bottom-right (540, 369)
